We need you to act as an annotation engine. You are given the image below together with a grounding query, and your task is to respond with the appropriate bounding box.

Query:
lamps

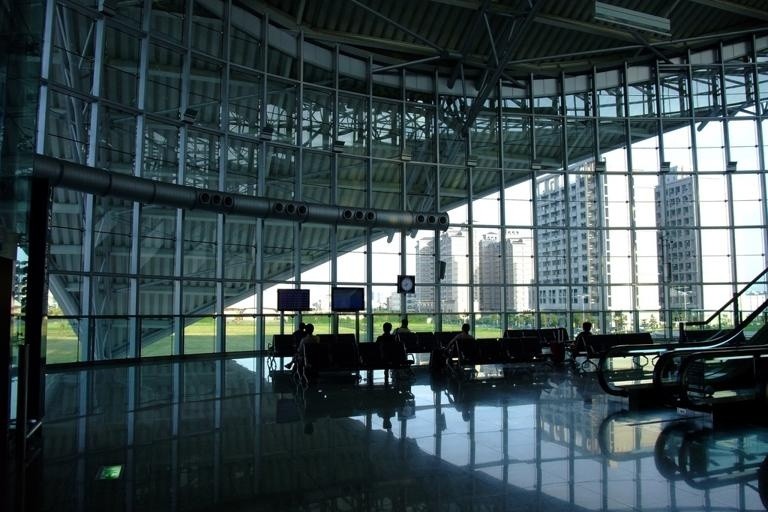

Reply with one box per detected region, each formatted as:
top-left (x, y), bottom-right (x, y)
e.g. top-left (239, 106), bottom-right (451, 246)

top-left (530, 158), bottom-right (543, 171)
top-left (333, 140), bottom-right (345, 153)
top-left (659, 161), bottom-right (671, 174)
top-left (726, 161), bottom-right (738, 172)
top-left (400, 149), bottom-right (412, 161)
top-left (466, 155), bottom-right (478, 167)
top-left (258, 126), bottom-right (274, 141)
top-left (594, 161), bottom-right (607, 173)
top-left (181, 105), bottom-right (198, 120)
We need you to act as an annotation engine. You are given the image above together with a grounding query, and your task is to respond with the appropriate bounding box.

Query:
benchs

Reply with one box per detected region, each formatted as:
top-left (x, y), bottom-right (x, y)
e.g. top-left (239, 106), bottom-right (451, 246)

top-left (679, 329), bottom-right (748, 345)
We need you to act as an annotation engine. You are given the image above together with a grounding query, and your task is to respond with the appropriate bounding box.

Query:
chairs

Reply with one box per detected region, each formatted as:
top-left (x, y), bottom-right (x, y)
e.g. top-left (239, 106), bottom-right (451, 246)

top-left (394, 327), bottom-right (657, 371)
top-left (265, 332), bottom-right (416, 403)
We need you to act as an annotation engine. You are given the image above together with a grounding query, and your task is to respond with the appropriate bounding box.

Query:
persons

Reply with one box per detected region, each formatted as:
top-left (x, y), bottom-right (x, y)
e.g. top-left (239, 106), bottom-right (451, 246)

top-left (374, 322), bottom-right (397, 344)
top-left (569, 321), bottom-right (593, 366)
top-left (284, 323), bottom-right (319, 369)
top-left (290, 322), bottom-right (307, 352)
top-left (394, 318), bottom-right (411, 336)
top-left (444, 323), bottom-right (476, 376)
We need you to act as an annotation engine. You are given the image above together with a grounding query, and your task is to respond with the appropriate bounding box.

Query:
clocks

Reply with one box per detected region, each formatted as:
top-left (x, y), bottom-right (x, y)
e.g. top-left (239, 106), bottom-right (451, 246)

top-left (397, 273), bottom-right (416, 295)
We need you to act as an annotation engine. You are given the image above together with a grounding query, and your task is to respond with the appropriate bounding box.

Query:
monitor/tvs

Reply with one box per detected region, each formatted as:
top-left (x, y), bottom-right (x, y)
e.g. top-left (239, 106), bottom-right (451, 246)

top-left (332, 287), bottom-right (365, 310)
top-left (277, 289), bottom-right (309, 310)
